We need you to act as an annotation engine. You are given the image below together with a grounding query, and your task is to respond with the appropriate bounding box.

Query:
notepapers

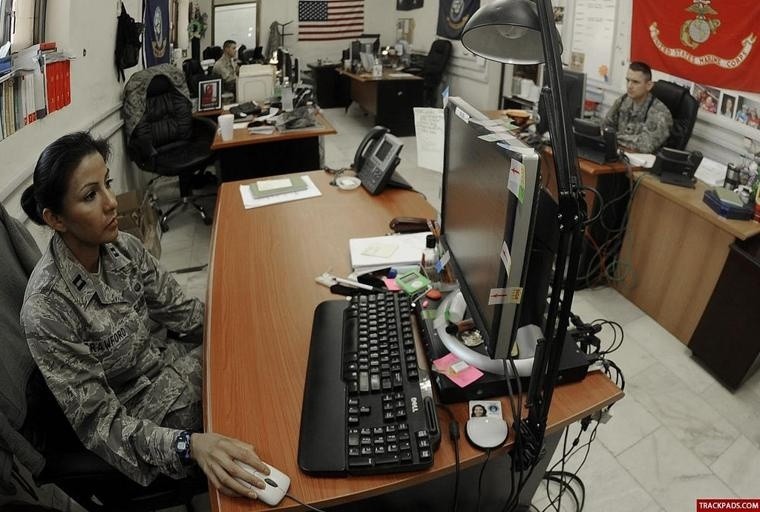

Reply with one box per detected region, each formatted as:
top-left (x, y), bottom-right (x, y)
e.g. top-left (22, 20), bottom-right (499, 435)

top-left (434, 250), bottom-right (452, 274)
top-left (499, 240), bottom-right (512, 280)
top-left (477, 133), bottom-right (517, 142)
top-left (496, 141), bottom-right (535, 156)
top-left (487, 287), bottom-right (524, 306)
top-left (505, 157), bottom-right (528, 205)
top-left (484, 123), bottom-right (520, 134)
top-left (482, 116), bottom-right (515, 125)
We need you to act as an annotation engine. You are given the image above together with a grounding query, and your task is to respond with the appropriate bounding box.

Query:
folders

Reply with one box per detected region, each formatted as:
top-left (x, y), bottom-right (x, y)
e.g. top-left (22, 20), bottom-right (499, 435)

top-left (22, 60), bottom-right (71, 126)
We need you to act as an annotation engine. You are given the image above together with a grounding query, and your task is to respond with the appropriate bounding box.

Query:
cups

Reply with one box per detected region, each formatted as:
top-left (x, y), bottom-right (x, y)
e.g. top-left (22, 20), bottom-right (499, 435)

top-left (218, 114), bottom-right (234, 141)
top-left (506, 112), bottom-right (531, 126)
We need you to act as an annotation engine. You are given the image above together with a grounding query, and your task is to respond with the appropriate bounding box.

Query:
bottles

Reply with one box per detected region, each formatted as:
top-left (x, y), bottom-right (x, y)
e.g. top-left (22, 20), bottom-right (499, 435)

top-left (281, 76), bottom-right (294, 112)
top-left (274, 77), bottom-right (280, 98)
top-left (723, 163), bottom-right (742, 192)
top-left (421, 234), bottom-right (439, 281)
top-left (306, 103), bottom-right (316, 124)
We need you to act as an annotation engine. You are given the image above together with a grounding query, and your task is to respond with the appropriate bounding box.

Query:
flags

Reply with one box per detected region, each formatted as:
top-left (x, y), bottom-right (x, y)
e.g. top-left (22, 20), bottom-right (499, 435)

top-left (298, 1), bottom-right (364, 41)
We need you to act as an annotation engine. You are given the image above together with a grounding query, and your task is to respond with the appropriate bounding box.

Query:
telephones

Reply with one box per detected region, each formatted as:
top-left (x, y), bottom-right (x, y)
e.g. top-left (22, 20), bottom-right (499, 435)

top-left (571, 118), bottom-right (619, 160)
top-left (293, 87), bottom-right (313, 109)
top-left (324, 125), bottom-right (404, 195)
top-left (652, 148), bottom-right (704, 180)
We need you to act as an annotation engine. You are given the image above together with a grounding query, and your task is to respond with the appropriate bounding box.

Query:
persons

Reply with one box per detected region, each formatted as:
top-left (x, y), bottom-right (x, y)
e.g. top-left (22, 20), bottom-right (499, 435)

top-left (746, 107), bottom-right (759, 128)
top-left (19, 134), bottom-right (270, 499)
top-left (212, 39), bottom-right (238, 82)
top-left (735, 104), bottom-right (749, 124)
top-left (603, 62), bottom-right (673, 154)
top-left (722, 97), bottom-right (732, 118)
top-left (702, 95), bottom-right (717, 114)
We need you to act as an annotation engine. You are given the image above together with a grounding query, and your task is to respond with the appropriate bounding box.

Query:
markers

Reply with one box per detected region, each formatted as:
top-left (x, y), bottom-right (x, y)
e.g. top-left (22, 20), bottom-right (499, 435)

top-left (445, 317), bottom-right (475, 336)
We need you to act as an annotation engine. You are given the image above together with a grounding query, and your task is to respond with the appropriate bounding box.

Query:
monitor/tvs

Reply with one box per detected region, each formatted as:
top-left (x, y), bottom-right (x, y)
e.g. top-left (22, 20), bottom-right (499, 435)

top-left (277, 46), bottom-right (292, 88)
top-left (535, 64), bottom-right (587, 130)
top-left (344, 34), bottom-right (362, 74)
top-left (436, 96), bottom-right (543, 361)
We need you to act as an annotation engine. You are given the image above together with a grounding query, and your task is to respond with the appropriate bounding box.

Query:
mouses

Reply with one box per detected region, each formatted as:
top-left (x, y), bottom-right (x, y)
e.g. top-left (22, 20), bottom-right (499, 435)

top-left (227, 457), bottom-right (292, 508)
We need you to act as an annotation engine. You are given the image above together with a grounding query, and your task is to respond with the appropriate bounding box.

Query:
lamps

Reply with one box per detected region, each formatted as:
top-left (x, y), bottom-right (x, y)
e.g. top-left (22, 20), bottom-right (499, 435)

top-left (459, 0), bottom-right (591, 469)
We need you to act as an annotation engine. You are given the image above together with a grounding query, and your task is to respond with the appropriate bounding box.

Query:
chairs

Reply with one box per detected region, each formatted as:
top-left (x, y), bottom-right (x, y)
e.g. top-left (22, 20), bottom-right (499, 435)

top-left (650, 80), bottom-right (697, 150)
top-left (2, 208), bottom-right (211, 512)
top-left (123, 64), bottom-right (218, 231)
top-left (183, 56), bottom-right (216, 99)
top-left (402, 40), bottom-right (453, 106)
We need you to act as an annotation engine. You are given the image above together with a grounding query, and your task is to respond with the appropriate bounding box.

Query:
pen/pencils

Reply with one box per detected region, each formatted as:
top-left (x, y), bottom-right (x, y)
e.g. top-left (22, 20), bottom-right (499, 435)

top-left (427, 217), bottom-right (455, 283)
top-left (333, 276), bottom-right (386, 294)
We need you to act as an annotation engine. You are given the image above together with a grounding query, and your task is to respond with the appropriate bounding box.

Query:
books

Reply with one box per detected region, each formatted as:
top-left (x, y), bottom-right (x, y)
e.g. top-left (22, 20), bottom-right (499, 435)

top-left (249, 175), bottom-right (308, 198)
top-left (715, 187), bottom-right (743, 207)
top-left (237, 175), bottom-right (322, 211)
top-left (349, 232), bottom-right (439, 274)
top-left (0, 42), bottom-right (76, 141)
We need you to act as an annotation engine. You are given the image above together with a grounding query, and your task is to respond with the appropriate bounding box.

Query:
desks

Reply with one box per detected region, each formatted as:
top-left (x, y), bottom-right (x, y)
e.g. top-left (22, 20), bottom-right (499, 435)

top-left (308, 59), bottom-right (352, 108)
top-left (336, 66), bottom-right (426, 121)
top-left (204, 162), bottom-right (622, 510)
top-left (612, 168), bottom-right (760, 391)
top-left (478, 110), bottom-right (647, 283)
top-left (211, 103), bottom-right (337, 179)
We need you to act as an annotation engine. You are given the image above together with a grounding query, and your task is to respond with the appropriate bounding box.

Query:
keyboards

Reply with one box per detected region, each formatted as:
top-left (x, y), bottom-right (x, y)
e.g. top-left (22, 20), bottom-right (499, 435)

top-left (296, 288), bottom-right (442, 481)
